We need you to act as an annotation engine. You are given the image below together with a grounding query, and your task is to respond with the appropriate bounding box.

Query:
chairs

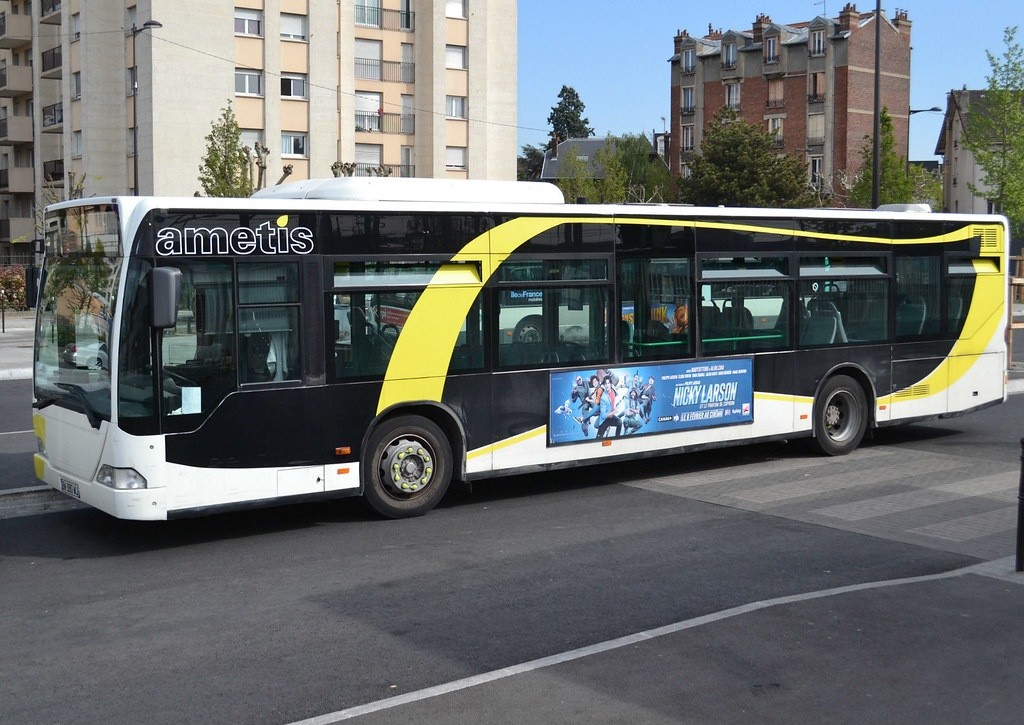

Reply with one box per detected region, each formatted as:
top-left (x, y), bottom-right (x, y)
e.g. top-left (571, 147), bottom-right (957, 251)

top-left (337, 281), bottom-right (964, 383)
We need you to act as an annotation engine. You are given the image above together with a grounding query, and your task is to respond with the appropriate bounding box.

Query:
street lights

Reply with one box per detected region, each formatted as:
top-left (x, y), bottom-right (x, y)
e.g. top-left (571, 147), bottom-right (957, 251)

top-left (906, 104), bottom-right (942, 177)
top-left (132, 19), bottom-right (163, 195)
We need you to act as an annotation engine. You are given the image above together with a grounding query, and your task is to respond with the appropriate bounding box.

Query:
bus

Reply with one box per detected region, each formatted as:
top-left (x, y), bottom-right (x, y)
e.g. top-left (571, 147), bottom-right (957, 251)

top-left (25, 176), bottom-right (1013, 522)
top-left (370, 264), bottom-right (820, 346)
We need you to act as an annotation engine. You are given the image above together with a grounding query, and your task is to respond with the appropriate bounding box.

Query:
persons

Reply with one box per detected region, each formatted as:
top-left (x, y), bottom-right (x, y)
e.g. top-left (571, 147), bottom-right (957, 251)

top-left (570, 369), bottom-right (661, 440)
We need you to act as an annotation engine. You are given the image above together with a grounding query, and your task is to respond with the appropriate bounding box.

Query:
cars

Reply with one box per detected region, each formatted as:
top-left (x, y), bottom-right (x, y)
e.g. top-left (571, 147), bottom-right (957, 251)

top-left (61, 338), bottom-right (110, 372)
